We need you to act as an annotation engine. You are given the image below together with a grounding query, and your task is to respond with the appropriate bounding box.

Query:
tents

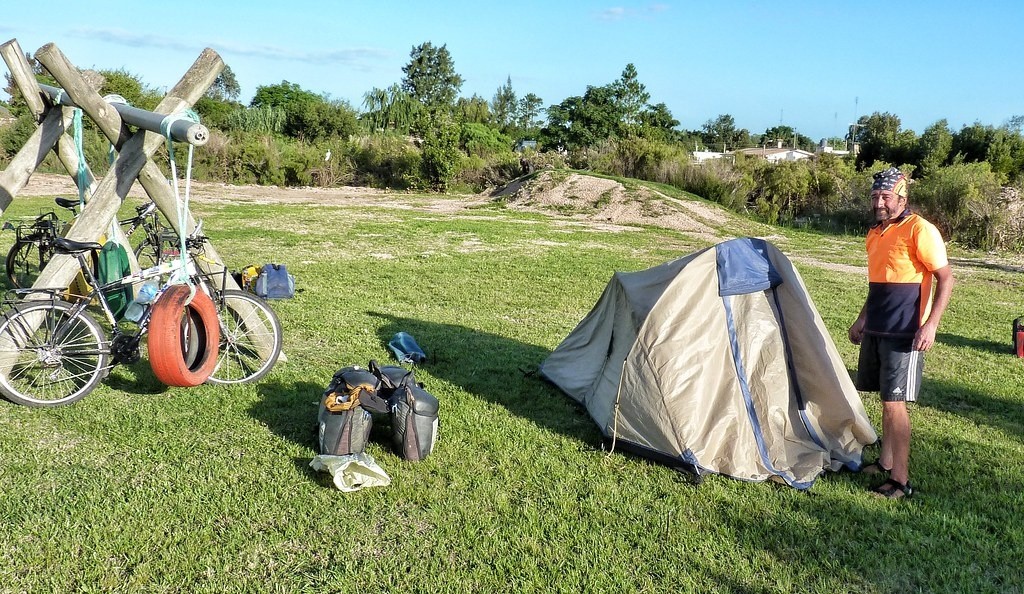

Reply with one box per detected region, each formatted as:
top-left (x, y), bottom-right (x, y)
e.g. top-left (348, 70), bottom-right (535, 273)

top-left (539, 238), bottom-right (878, 491)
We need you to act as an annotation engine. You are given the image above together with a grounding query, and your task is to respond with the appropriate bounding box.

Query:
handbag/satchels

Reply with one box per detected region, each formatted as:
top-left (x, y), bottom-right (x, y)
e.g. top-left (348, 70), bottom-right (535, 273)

top-left (309, 451), bottom-right (391, 492)
top-left (317, 360), bottom-right (439, 462)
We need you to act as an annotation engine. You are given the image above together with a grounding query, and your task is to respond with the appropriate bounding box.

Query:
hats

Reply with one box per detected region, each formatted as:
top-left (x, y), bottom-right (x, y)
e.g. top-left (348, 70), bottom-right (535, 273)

top-left (871, 167), bottom-right (909, 198)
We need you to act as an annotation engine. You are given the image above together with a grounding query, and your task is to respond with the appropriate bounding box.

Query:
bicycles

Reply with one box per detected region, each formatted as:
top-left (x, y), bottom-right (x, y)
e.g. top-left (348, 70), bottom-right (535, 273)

top-left (5, 196), bottom-right (174, 299)
top-left (0, 218), bottom-right (283, 408)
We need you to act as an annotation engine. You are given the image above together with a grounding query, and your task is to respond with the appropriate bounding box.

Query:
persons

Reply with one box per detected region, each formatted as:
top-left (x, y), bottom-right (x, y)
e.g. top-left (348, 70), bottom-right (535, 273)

top-left (848, 167), bottom-right (953, 499)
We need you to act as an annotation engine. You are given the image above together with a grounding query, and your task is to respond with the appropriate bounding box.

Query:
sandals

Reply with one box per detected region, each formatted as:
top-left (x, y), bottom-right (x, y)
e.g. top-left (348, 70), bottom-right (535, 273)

top-left (874, 479), bottom-right (913, 501)
top-left (860, 458), bottom-right (892, 476)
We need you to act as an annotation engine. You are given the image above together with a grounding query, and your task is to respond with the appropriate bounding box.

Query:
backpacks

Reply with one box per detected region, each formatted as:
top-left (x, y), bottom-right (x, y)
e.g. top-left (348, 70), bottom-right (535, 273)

top-left (257, 264), bottom-right (295, 299)
